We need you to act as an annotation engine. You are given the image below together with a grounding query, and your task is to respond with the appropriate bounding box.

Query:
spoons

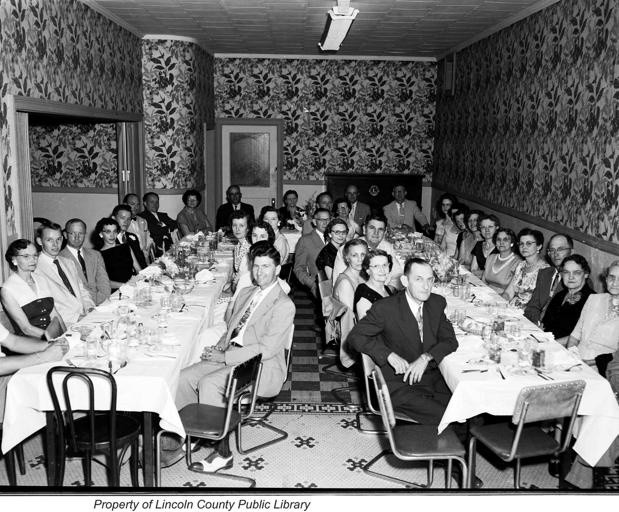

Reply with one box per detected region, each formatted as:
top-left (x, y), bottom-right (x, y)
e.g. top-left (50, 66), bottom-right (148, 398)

top-left (114, 360), bottom-right (127, 374)
top-left (179, 304), bottom-right (185, 312)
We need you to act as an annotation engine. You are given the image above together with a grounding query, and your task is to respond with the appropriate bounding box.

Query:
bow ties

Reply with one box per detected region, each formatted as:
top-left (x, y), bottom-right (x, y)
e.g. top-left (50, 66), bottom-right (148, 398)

top-left (131, 217), bottom-right (136, 221)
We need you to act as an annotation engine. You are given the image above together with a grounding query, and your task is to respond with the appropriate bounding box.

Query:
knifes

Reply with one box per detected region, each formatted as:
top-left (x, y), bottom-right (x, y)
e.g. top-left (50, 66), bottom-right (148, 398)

top-left (64, 358), bottom-right (74, 366)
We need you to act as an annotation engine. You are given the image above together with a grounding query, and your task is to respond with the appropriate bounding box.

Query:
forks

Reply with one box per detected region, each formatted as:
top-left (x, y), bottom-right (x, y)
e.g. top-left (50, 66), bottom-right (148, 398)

top-left (109, 360), bottom-right (113, 375)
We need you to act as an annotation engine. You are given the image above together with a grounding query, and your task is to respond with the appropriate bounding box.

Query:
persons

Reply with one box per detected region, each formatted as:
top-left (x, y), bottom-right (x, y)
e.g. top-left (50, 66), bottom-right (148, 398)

top-left (122, 192), bottom-right (156, 265)
top-left (0, 322), bottom-right (68, 375)
top-left (110, 204), bottom-right (149, 271)
top-left (314, 191), bottom-right (336, 221)
top-left (175, 244), bottom-right (297, 474)
top-left (352, 249), bottom-right (399, 323)
top-left (315, 217), bottom-right (349, 279)
top-left (332, 197), bottom-right (356, 243)
top-left (277, 188), bottom-right (306, 229)
top-left (542, 258), bottom-right (619, 479)
top-left (29, 221), bottom-right (98, 329)
top-left (0, 237), bottom-right (68, 342)
top-left (293, 207), bottom-right (332, 294)
top-left (176, 188), bottom-right (213, 237)
top-left (382, 183), bottom-right (434, 236)
top-left (434, 192), bottom-right (598, 346)
top-left (246, 220), bottom-right (275, 245)
top-left (220, 208), bottom-right (253, 298)
top-left (136, 193), bottom-right (182, 257)
top-left (94, 215), bottom-right (142, 293)
top-left (345, 258), bottom-right (484, 490)
top-left (332, 212), bottom-right (404, 296)
top-left (344, 184), bottom-right (373, 227)
top-left (332, 238), bottom-right (369, 313)
top-left (258, 205), bottom-right (291, 266)
top-left (187, 240), bottom-right (291, 371)
top-left (59, 218), bottom-right (112, 306)
top-left (215, 184), bottom-right (257, 231)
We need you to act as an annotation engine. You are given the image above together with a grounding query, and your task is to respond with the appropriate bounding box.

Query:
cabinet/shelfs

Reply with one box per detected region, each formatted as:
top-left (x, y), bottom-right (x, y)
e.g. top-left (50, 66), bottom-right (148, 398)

top-left (324, 172), bottom-right (425, 232)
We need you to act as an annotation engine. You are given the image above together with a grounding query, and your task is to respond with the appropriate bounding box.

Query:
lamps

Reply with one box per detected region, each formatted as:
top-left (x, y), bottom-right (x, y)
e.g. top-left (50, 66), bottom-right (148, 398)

top-left (317, 0), bottom-right (359, 52)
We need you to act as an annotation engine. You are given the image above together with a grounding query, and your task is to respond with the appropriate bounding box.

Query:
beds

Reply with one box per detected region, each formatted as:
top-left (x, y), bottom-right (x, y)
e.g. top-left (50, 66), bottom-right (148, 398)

top-left (4, 226), bottom-right (612, 493)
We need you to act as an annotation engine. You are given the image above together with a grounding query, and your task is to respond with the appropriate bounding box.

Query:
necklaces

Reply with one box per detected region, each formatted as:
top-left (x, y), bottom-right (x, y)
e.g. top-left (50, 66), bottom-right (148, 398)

top-left (26, 278), bottom-right (34, 287)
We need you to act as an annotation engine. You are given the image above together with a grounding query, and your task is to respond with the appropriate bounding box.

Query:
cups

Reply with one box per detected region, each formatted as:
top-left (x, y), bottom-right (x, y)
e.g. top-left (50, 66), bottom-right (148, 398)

top-left (533, 341), bottom-right (548, 369)
top-left (157, 314), bottom-right (167, 334)
top-left (489, 335), bottom-right (502, 362)
top-left (455, 308), bottom-right (467, 331)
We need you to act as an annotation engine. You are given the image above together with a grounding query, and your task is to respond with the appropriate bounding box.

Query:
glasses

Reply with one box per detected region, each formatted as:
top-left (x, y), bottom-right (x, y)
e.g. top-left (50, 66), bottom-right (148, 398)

top-left (518, 240), bottom-right (537, 246)
top-left (562, 269), bottom-right (582, 276)
top-left (331, 228), bottom-right (348, 235)
top-left (547, 247), bottom-right (570, 253)
top-left (103, 229), bottom-right (117, 233)
top-left (316, 217), bottom-right (331, 222)
top-left (495, 237), bottom-right (512, 241)
top-left (417, 276), bottom-right (437, 282)
top-left (371, 262), bottom-right (389, 269)
top-left (67, 231), bottom-right (84, 235)
top-left (478, 225), bottom-right (492, 229)
top-left (16, 254), bottom-right (38, 258)
top-left (606, 275), bottom-right (619, 282)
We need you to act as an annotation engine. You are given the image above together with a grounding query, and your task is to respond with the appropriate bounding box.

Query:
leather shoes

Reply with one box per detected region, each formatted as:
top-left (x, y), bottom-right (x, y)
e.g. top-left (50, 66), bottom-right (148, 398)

top-left (188, 450), bottom-right (234, 473)
top-left (450, 466), bottom-right (485, 491)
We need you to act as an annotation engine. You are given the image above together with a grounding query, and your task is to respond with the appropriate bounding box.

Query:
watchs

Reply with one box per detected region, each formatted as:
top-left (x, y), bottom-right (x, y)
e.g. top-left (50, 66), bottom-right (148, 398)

top-left (420, 353), bottom-right (432, 364)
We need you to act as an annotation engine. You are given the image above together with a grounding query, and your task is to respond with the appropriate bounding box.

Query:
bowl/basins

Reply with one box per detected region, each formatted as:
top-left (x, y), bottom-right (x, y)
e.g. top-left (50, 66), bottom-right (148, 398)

top-left (173, 283), bottom-right (196, 295)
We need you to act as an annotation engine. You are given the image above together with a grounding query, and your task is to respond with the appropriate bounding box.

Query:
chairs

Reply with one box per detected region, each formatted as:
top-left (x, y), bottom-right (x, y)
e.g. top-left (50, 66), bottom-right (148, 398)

top-left (48, 367), bottom-right (141, 487)
top-left (236, 325), bottom-right (295, 454)
top-left (353, 318), bottom-right (389, 435)
top-left (322, 296), bottom-right (354, 381)
top-left (316, 280), bottom-right (354, 379)
top-left (156, 353), bottom-right (264, 487)
top-left (356, 352), bottom-right (433, 487)
top-left (468, 384), bottom-right (584, 491)
top-left (369, 372), bottom-right (468, 489)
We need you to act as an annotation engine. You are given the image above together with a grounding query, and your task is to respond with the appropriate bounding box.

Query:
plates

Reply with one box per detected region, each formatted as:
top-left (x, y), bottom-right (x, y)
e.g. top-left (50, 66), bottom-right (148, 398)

top-left (109, 295), bottom-right (130, 302)
top-left (70, 323), bottom-right (96, 332)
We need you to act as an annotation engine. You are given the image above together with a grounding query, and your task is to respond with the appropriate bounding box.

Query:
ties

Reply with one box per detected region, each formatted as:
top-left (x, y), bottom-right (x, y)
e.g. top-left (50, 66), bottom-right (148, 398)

top-left (77, 250), bottom-right (88, 280)
top-left (417, 307), bottom-right (423, 342)
top-left (399, 203), bottom-right (405, 215)
top-left (230, 290), bottom-right (264, 340)
top-left (550, 271), bottom-right (560, 292)
top-left (122, 235), bottom-right (125, 243)
top-left (349, 205), bottom-right (355, 220)
top-left (235, 206), bottom-right (237, 211)
top-left (53, 259), bottom-right (76, 296)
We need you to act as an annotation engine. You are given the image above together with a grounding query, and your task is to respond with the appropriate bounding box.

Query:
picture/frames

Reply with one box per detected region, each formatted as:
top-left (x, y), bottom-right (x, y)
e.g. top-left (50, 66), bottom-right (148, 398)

top-left (442, 51), bottom-right (456, 96)
